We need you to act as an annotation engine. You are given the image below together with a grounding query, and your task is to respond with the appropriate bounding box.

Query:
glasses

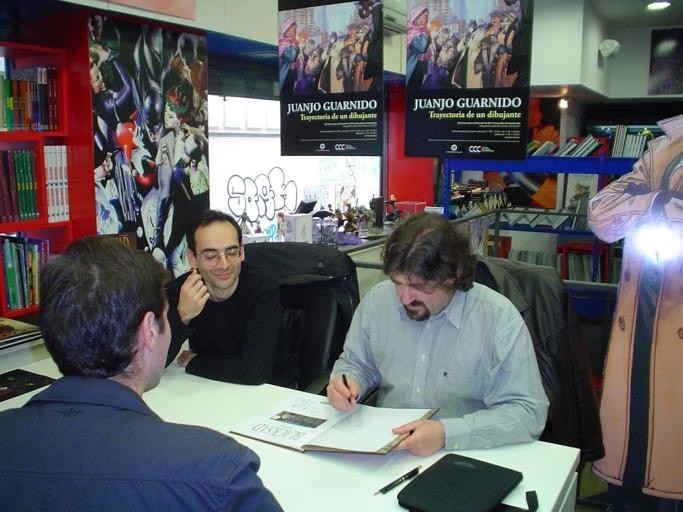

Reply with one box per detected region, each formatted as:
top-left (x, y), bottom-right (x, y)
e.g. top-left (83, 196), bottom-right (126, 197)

top-left (196, 249), bottom-right (241, 264)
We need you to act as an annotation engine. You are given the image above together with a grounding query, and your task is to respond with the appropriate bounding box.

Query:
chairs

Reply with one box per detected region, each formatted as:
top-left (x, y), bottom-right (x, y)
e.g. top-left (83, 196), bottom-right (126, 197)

top-left (360, 254), bottom-right (607, 495)
top-left (242, 240), bottom-right (360, 395)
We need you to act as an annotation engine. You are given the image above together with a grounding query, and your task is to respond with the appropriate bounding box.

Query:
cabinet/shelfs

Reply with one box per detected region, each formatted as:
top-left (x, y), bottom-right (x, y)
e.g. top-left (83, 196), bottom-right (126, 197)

top-left (0, 0), bottom-right (99, 317)
top-left (444, 155), bottom-right (642, 323)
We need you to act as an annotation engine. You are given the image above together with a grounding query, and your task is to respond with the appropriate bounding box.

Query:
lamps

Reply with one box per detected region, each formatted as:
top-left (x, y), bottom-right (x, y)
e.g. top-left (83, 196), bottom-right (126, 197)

top-left (599, 39), bottom-right (621, 59)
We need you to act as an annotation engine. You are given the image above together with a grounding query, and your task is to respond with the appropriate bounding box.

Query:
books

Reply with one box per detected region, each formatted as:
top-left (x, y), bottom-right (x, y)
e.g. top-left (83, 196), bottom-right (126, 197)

top-left (507, 124), bottom-right (648, 284)
top-left (0, 57), bottom-right (69, 350)
top-left (227, 394), bottom-right (440, 455)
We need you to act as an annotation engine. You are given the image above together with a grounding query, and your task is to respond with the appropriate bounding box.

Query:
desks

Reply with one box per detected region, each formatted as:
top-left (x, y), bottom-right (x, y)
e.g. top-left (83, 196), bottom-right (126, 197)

top-left (0, 344), bottom-right (581, 512)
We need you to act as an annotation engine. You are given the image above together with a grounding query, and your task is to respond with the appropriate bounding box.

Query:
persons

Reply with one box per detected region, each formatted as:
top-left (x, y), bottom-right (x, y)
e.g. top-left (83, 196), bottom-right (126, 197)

top-left (407, 0), bottom-right (531, 91)
top-left (0, 236), bottom-right (284, 512)
top-left (278, 0), bottom-right (383, 97)
top-left (163, 210), bottom-right (303, 390)
top-left (326, 212), bottom-right (551, 459)
top-left (90, 42), bottom-right (210, 280)
top-left (587, 113), bottom-right (683, 512)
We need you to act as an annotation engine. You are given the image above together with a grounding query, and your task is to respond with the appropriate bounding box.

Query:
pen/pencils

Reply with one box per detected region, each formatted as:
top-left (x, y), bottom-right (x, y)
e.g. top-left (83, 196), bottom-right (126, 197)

top-left (376, 467), bottom-right (421, 496)
top-left (343, 373), bottom-right (353, 406)
